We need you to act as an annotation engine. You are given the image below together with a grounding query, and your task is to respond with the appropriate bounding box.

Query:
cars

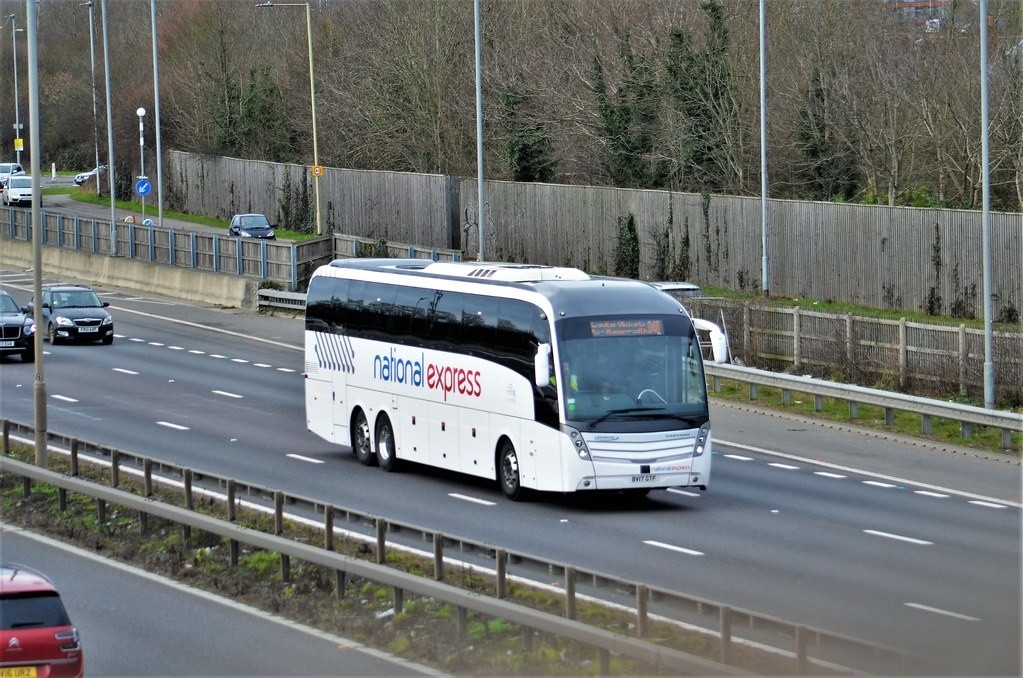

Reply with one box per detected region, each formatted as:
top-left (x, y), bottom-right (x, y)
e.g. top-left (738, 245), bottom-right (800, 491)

top-left (227, 213), bottom-right (277, 242)
top-left (74, 164), bottom-right (104, 187)
top-left (649, 280), bottom-right (703, 297)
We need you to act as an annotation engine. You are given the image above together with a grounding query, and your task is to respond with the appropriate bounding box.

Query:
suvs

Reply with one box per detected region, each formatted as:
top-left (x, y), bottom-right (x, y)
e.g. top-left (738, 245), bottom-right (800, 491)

top-left (1, 561), bottom-right (85, 678)
top-left (2, 175), bottom-right (43, 207)
top-left (27, 282), bottom-right (115, 345)
top-left (0, 290), bottom-right (35, 364)
top-left (0, 161), bottom-right (26, 190)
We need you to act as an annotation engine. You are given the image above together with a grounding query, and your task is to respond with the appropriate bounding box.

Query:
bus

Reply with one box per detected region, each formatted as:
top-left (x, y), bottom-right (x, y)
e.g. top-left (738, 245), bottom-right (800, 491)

top-left (301, 256), bottom-right (728, 504)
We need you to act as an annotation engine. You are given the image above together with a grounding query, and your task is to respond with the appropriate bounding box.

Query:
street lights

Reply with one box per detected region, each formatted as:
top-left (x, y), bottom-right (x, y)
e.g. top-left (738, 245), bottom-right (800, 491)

top-left (253, 0), bottom-right (323, 236)
